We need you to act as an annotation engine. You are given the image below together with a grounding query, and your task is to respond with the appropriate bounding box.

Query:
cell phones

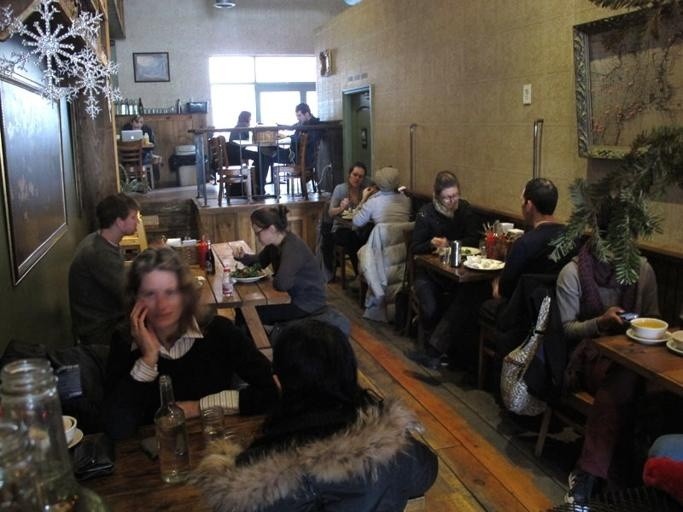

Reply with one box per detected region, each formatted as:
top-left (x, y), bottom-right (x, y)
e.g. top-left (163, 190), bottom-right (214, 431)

top-left (620, 310), bottom-right (639, 322)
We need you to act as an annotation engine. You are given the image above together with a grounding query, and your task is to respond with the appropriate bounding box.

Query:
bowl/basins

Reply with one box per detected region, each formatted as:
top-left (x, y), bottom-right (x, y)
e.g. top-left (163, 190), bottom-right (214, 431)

top-left (63, 416), bottom-right (77, 443)
top-left (630, 318), bottom-right (668, 340)
top-left (672, 331), bottom-right (683, 349)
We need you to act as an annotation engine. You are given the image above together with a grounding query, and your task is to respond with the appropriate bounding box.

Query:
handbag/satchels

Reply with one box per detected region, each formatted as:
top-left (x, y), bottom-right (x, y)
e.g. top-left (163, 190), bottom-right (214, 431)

top-left (499, 290), bottom-right (552, 418)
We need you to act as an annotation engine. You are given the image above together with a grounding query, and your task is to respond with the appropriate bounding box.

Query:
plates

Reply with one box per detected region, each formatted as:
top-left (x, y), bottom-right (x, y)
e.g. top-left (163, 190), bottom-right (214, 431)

top-left (667, 341), bottom-right (683, 353)
top-left (342, 209), bottom-right (357, 215)
top-left (232, 266), bottom-right (267, 283)
top-left (343, 215), bottom-right (354, 219)
top-left (464, 259), bottom-right (504, 270)
top-left (67, 428), bottom-right (83, 449)
top-left (627, 329), bottom-right (671, 343)
top-left (447, 247), bottom-right (481, 256)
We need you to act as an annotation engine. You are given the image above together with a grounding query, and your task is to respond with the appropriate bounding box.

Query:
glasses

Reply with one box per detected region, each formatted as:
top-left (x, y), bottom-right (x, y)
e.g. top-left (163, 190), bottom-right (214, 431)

top-left (437, 191), bottom-right (462, 201)
top-left (255, 228), bottom-right (264, 236)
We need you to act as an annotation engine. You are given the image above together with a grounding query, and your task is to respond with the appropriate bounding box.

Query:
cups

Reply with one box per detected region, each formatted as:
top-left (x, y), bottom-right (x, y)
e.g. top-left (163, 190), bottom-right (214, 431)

top-left (199, 407), bottom-right (224, 446)
top-left (438, 247), bottom-right (448, 265)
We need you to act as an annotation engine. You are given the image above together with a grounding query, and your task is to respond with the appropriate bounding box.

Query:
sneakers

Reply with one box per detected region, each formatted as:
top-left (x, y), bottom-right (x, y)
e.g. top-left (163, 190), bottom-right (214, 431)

top-left (415, 346), bottom-right (444, 381)
top-left (563, 468), bottom-right (597, 512)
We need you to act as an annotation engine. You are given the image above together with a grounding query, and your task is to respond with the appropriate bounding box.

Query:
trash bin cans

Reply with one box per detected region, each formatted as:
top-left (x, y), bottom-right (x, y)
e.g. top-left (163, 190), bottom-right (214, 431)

top-left (168, 144), bottom-right (199, 186)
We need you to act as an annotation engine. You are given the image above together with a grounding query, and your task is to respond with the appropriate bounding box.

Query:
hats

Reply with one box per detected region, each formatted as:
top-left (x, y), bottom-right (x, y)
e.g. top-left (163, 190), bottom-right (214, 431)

top-left (375, 166), bottom-right (400, 192)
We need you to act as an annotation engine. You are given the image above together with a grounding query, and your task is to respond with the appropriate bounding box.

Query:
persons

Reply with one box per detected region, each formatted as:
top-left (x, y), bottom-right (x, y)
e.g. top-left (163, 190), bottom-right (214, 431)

top-left (328, 163), bottom-right (370, 275)
top-left (80, 245), bottom-right (281, 434)
top-left (194, 320), bottom-right (439, 512)
top-left (233, 205), bottom-right (327, 325)
top-left (411, 171), bottom-right (486, 348)
top-left (121, 114), bottom-right (155, 191)
top-left (68, 193), bottom-right (139, 343)
top-left (226, 111), bottom-right (260, 196)
top-left (555, 201), bottom-right (659, 512)
top-left (425, 178), bottom-right (583, 358)
top-left (254, 102), bottom-right (321, 195)
top-left (353, 167), bottom-right (411, 323)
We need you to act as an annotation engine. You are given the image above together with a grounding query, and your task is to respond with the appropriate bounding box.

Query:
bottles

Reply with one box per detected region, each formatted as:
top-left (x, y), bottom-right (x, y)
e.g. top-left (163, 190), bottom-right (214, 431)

top-left (205, 240), bottom-right (215, 275)
top-left (143, 132), bottom-right (149, 145)
top-left (155, 376), bottom-right (191, 482)
top-left (198, 234), bottom-right (208, 270)
top-left (451, 240), bottom-right (461, 268)
top-left (116, 96), bottom-right (144, 115)
top-left (0, 359), bottom-right (109, 512)
top-left (222, 268), bottom-right (233, 294)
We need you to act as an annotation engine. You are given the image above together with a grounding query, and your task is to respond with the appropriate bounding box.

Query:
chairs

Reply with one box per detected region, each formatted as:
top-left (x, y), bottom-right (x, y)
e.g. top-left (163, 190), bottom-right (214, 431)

top-left (359, 222), bottom-right (416, 311)
top-left (323, 201), bottom-right (348, 289)
top-left (404, 229), bottom-right (426, 337)
top-left (117, 138), bottom-right (147, 183)
top-left (211, 135), bottom-right (257, 207)
top-left (272, 132), bottom-right (320, 201)
top-left (476, 273), bottom-right (559, 389)
top-left (533, 286), bottom-right (596, 458)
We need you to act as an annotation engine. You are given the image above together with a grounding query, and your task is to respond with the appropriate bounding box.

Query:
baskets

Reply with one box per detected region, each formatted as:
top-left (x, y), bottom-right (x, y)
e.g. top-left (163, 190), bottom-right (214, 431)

top-left (171, 244), bottom-right (199, 265)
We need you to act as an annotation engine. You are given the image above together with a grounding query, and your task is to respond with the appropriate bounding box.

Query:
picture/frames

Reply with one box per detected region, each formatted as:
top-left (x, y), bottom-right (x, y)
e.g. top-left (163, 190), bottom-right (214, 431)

top-left (132, 52), bottom-right (170, 83)
top-left (318, 48), bottom-right (333, 77)
top-left (0, 65), bottom-right (70, 287)
top-left (571, 1), bottom-right (683, 161)
